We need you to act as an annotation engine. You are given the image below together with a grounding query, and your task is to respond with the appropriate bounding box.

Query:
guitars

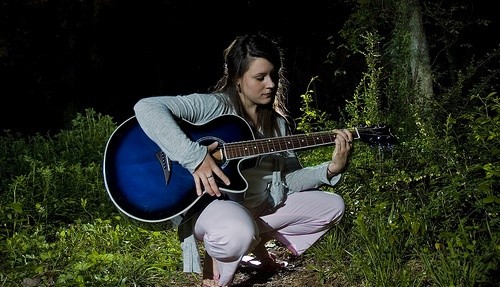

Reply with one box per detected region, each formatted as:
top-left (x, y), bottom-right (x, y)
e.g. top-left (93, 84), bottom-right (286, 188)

top-left (102, 114), bottom-right (400, 231)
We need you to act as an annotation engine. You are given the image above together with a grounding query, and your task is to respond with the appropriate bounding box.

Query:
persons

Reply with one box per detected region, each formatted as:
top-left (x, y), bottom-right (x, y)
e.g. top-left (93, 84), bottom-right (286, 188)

top-left (133, 33), bottom-right (353, 287)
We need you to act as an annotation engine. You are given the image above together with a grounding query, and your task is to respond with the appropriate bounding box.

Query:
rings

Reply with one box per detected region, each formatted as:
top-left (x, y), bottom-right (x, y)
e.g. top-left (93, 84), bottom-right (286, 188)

top-left (346, 140), bottom-right (350, 143)
top-left (206, 175), bottom-right (213, 179)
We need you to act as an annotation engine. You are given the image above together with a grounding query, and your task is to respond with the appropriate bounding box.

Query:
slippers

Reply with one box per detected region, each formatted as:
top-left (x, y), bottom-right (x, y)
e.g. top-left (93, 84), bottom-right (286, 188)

top-left (203, 269), bottom-right (222, 287)
top-left (241, 253), bottom-right (288, 270)
